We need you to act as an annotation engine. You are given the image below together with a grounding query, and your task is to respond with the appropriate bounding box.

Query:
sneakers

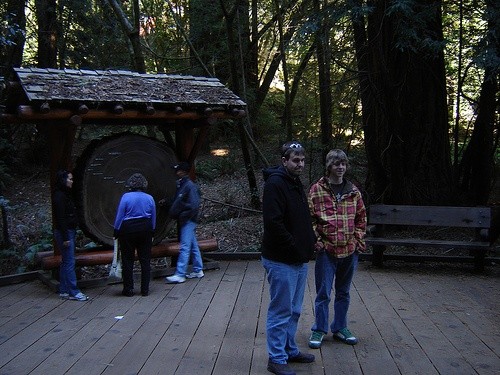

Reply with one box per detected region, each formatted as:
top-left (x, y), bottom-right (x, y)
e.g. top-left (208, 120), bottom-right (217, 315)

top-left (68, 292), bottom-right (90, 301)
top-left (308, 331), bottom-right (326, 349)
top-left (165, 275), bottom-right (187, 284)
top-left (59, 292), bottom-right (70, 297)
top-left (186, 270), bottom-right (204, 279)
top-left (333, 327), bottom-right (359, 345)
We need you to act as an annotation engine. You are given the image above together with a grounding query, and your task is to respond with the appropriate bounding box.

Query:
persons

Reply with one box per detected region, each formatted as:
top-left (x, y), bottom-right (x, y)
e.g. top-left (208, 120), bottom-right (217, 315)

top-left (260, 142), bottom-right (318, 375)
top-left (308, 149), bottom-right (367, 348)
top-left (165, 162), bottom-right (205, 283)
top-left (51, 168), bottom-right (90, 301)
top-left (112, 173), bottom-right (157, 296)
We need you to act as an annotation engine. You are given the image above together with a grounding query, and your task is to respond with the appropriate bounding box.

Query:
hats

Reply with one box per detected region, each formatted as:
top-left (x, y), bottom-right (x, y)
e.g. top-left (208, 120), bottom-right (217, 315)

top-left (170, 162), bottom-right (187, 170)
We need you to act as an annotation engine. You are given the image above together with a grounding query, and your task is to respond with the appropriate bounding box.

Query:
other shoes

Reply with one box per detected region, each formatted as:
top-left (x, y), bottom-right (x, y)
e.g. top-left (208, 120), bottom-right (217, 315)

top-left (140, 291), bottom-right (148, 296)
top-left (122, 290), bottom-right (134, 297)
top-left (267, 358), bottom-right (297, 375)
top-left (288, 352), bottom-right (315, 363)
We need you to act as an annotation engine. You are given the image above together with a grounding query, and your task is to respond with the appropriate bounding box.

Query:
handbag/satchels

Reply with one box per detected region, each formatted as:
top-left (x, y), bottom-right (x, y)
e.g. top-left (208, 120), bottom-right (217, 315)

top-left (108, 238), bottom-right (123, 282)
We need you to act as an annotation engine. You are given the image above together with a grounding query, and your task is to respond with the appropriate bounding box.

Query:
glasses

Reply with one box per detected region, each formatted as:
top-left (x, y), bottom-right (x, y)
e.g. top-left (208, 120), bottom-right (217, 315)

top-left (283, 143), bottom-right (302, 157)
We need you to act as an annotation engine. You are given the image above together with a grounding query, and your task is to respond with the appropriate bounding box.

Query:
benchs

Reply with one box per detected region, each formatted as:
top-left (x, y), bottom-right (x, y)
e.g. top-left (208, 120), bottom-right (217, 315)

top-left (365, 204), bottom-right (491, 272)
